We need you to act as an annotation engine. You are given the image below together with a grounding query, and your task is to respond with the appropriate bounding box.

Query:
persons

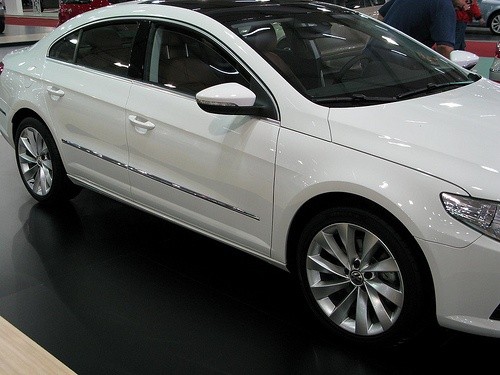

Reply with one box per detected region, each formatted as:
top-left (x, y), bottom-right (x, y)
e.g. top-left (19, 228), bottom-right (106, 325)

top-left (0, 0), bottom-right (7, 34)
top-left (360, 0), bottom-right (456, 69)
top-left (455, 0), bottom-right (486, 50)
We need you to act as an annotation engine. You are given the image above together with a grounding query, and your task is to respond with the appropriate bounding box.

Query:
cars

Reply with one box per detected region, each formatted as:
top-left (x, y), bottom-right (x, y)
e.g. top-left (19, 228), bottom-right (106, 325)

top-left (1, 1), bottom-right (499, 350)
top-left (458, 1), bottom-right (500, 35)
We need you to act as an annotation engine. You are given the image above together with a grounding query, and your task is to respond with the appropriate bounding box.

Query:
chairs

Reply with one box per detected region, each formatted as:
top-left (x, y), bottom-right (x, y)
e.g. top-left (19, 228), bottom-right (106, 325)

top-left (81, 28), bottom-right (133, 71)
top-left (155, 28), bottom-right (210, 87)
top-left (236, 24), bottom-right (292, 86)
